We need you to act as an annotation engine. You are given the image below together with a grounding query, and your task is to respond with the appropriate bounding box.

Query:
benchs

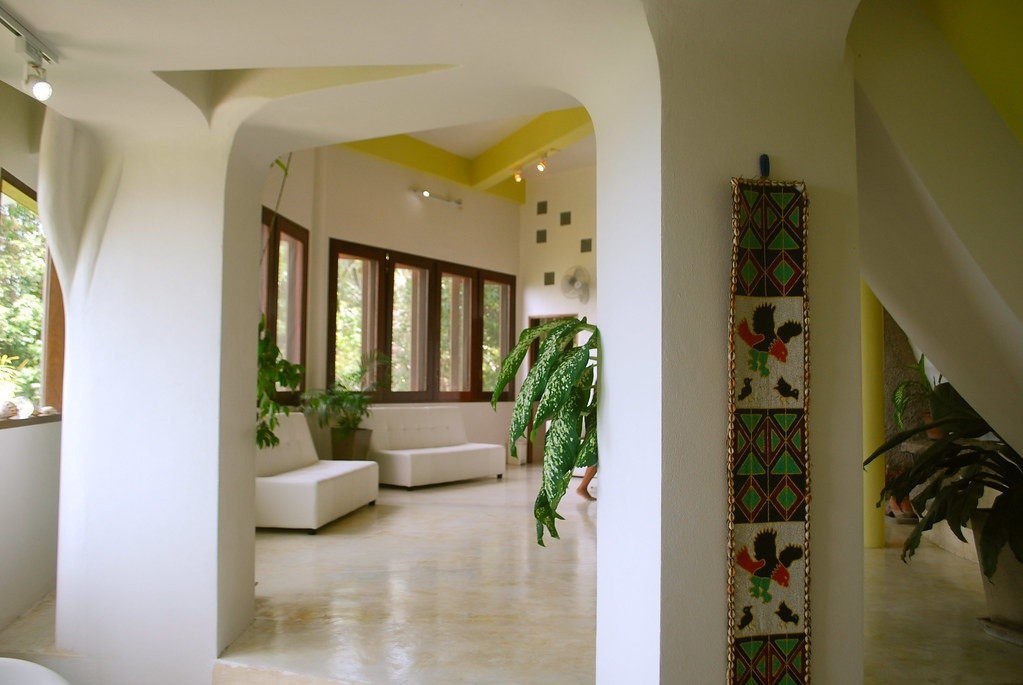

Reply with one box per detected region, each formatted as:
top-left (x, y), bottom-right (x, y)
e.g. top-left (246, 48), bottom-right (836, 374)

top-left (359, 407), bottom-right (506, 490)
top-left (256, 412), bottom-right (380, 535)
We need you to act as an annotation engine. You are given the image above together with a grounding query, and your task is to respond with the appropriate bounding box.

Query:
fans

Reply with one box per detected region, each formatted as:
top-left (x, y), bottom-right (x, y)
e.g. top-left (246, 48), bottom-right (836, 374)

top-left (561, 265), bottom-right (590, 303)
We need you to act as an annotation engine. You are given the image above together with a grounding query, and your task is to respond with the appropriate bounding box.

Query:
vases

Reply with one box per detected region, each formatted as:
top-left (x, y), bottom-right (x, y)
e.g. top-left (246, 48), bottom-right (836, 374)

top-left (887, 468), bottom-right (915, 518)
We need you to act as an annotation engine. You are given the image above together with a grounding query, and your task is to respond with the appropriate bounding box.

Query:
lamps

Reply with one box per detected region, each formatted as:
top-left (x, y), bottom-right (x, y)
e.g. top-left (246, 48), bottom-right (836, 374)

top-left (10, 35), bottom-right (53, 102)
top-left (537, 158), bottom-right (550, 171)
top-left (513, 169), bottom-right (522, 182)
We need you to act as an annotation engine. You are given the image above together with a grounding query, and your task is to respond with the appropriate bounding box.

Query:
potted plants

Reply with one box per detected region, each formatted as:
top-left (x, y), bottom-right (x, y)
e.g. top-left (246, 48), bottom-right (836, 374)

top-left (863, 383), bottom-right (1023, 630)
top-left (297, 348), bottom-right (404, 462)
top-left (893, 353), bottom-right (948, 439)
